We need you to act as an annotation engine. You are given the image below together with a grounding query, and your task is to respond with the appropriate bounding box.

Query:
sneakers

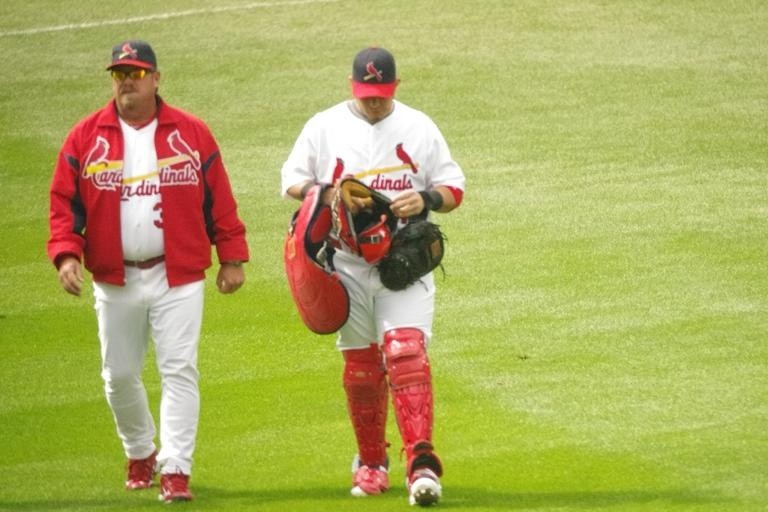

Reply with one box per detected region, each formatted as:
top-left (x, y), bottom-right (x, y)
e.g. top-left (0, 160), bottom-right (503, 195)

top-left (158, 474), bottom-right (194, 505)
top-left (126, 448), bottom-right (161, 490)
top-left (352, 455), bottom-right (389, 497)
top-left (406, 468), bottom-right (442, 505)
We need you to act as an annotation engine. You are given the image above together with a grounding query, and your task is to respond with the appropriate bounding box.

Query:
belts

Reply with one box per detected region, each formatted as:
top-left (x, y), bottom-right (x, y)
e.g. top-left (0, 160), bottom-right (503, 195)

top-left (123, 255), bottom-right (165, 269)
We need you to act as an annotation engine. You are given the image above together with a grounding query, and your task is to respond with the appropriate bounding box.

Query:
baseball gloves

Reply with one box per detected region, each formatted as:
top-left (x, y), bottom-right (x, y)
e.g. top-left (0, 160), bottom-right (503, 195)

top-left (377, 220), bottom-right (443, 292)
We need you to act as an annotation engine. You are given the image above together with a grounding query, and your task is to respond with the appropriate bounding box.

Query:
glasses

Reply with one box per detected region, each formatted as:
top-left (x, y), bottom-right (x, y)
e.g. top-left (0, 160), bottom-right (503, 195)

top-left (110, 68), bottom-right (147, 80)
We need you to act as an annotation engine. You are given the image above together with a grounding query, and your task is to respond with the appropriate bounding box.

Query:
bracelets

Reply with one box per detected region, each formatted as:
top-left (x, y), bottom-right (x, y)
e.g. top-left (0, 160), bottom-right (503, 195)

top-left (420, 188), bottom-right (444, 212)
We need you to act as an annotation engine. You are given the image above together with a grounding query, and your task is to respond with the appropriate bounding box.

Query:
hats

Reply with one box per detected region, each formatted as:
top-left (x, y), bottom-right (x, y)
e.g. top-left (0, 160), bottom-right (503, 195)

top-left (352, 48), bottom-right (396, 100)
top-left (105, 39), bottom-right (157, 70)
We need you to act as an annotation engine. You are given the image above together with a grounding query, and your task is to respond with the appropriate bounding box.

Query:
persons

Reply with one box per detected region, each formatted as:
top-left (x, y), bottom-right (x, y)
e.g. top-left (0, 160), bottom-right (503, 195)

top-left (47, 41), bottom-right (250, 504)
top-left (279, 46), bottom-right (469, 507)
